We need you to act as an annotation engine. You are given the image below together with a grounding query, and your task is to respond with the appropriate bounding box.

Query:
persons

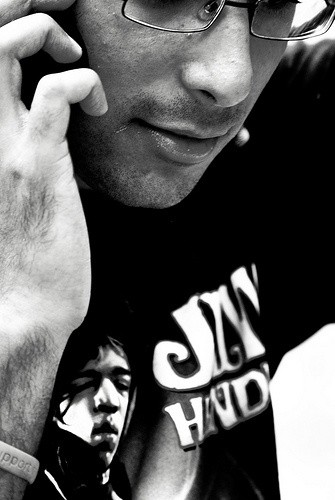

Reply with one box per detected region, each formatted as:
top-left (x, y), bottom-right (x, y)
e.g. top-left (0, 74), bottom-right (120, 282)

top-left (0, 0), bottom-right (334, 500)
top-left (30, 327), bottom-right (147, 499)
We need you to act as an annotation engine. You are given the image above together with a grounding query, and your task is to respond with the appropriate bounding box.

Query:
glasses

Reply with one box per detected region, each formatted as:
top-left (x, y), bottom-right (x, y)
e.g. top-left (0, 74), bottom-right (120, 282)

top-left (121, 0), bottom-right (335, 41)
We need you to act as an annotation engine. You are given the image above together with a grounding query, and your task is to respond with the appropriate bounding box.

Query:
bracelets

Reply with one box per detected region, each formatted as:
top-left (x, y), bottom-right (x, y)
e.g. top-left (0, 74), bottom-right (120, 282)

top-left (1, 439), bottom-right (42, 485)
